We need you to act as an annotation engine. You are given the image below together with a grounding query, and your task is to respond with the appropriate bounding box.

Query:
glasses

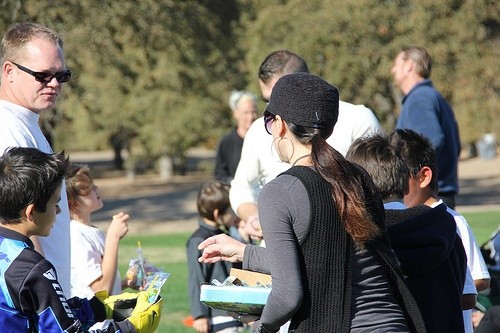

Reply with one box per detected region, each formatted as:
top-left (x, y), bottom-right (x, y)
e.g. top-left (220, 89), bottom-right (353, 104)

top-left (263, 113), bottom-right (275, 134)
top-left (10, 61), bottom-right (72, 83)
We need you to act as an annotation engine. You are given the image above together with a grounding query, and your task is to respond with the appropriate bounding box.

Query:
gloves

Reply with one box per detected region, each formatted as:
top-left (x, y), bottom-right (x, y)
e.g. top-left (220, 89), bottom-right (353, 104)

top-left (94, 289), bottom-right (163, 333)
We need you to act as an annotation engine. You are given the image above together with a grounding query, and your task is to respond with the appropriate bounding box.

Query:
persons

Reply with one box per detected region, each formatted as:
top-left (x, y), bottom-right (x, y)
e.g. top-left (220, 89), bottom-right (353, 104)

top-left (185, 179), bottom-right (246, 333)
top-left (214, 89), bottom-right (259, 180)
top-left (0, 22), bottom-right (72, 300)
top-left (384, 129), bottom-right (491, 333)
top-left (64, 163), bottom-right (130, 301)
top-left (344, 127), bottom-right (478, 333)
top-left (0, 146), bottom-right (162, 333)
top-left (228, 50), bottom-right (384, 247)
top-left (390, 45), bottom-right (461, 210)
top-left (197, 76), bottom-right (427, 333)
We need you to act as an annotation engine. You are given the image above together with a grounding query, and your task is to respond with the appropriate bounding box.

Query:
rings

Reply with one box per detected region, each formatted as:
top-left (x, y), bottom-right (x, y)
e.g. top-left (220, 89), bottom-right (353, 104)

top-left (218, 253), bottom-right (220, 256)
top-left (238, 313), bottom-right (244, 322)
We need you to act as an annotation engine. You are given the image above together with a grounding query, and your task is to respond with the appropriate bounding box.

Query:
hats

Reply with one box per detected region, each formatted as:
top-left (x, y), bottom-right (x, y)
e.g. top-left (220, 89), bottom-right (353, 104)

top-left (265, 73), bottom-right (339, 130)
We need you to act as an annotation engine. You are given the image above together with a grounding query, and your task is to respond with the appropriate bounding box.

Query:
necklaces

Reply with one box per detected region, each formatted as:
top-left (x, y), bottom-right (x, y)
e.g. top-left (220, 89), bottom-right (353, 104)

top-left (293, 155), bottom-right (310, 166)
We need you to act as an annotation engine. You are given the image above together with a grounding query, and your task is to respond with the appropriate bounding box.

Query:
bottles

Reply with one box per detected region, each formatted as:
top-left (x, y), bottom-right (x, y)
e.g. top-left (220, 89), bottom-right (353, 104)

top-left (250, 176), bottom-right (263, 203)
